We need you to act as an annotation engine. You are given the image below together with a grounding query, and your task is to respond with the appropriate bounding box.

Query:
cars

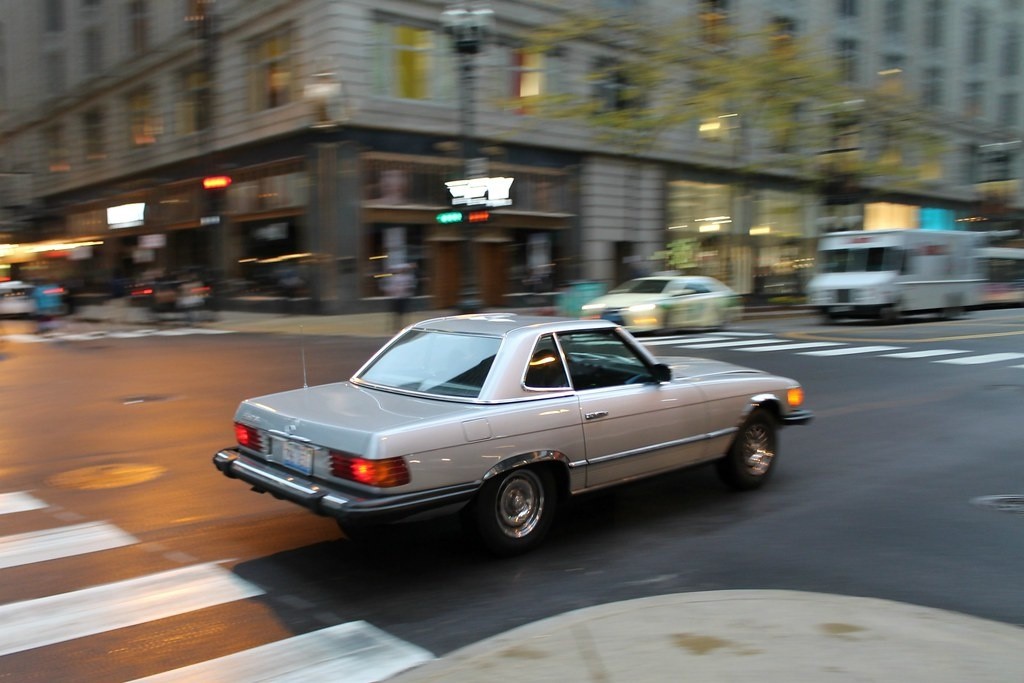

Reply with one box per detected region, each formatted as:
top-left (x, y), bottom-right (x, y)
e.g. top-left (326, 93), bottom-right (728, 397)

top-left (581, 275), bottom-right (743, 338)
top-left (212, 313), bottom-right (814, 561)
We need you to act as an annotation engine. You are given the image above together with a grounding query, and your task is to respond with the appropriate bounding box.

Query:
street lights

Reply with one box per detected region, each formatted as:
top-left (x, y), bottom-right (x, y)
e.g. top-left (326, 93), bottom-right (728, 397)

top-left (441, 4), bottom-right (493, 314)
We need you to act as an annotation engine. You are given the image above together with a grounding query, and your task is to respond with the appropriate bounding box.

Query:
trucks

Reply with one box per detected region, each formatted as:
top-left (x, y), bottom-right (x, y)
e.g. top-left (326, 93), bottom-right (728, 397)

top-left (806, 229), bottom-right (988, 326)
